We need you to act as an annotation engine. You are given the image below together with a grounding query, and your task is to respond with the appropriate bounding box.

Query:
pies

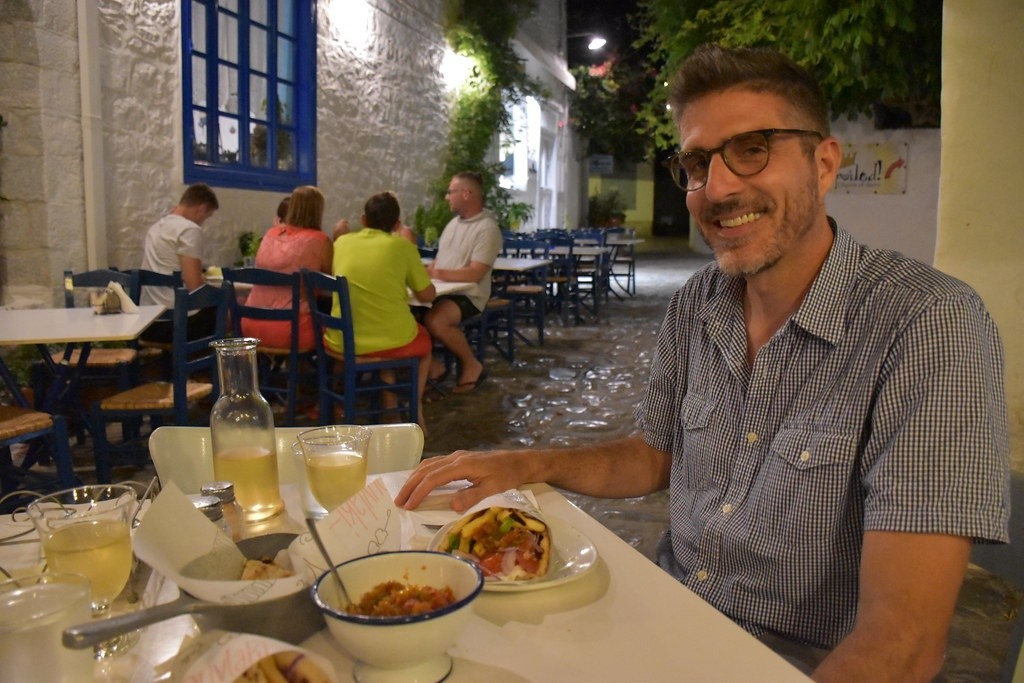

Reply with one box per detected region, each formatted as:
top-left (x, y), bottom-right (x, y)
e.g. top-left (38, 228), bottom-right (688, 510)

top-left (234, 651), bottom-right (333, 683)
top-left (437, 507), bottom-right (549, 582)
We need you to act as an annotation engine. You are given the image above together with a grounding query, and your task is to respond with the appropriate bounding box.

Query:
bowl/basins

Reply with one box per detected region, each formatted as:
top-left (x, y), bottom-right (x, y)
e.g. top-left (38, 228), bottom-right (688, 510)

top-left (311, 550), bottom-right (485, 683)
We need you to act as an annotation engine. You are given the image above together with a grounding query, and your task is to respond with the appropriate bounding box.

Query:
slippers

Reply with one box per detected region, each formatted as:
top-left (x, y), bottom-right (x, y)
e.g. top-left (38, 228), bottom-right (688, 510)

top-left (451, 365), bottom-right (484, 395)
top-left (423, 371), bottom-right (449, 392)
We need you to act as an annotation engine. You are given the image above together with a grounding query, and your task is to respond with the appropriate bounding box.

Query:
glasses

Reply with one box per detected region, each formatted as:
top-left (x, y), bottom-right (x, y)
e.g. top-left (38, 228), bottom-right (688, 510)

top-left (671, 129), bottom-right (824, 192)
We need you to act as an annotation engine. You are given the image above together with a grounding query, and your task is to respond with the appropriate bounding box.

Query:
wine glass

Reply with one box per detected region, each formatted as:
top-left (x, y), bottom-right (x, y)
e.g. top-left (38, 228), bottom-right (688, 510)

top-left (27, 485), bottom-right (140, 665)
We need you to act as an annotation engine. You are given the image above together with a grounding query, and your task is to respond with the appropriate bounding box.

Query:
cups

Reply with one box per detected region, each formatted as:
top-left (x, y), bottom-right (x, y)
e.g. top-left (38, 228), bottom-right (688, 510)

top-left (291, 441), bottom-right (329, 520)
top-left (297, 424), bottom-right (373, 513)
top-left (0, 570), bottom-right (97, 683)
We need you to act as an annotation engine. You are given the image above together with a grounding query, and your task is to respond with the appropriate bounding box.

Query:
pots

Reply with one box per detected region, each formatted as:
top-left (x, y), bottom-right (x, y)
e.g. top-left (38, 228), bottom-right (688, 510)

top-left (62, 532), bottom-right (328, 651)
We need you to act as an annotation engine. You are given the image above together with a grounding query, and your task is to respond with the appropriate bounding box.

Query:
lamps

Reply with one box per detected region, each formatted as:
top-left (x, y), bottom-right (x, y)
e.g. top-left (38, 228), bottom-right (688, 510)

top-left (556, 32), bottom-right (607, 55)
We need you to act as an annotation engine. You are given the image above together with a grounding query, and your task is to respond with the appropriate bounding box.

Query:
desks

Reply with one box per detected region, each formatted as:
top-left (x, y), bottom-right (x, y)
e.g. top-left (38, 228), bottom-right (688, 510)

top-left (418, 256), bottom-right (552, 361)
top-left (407, 280), bottom-right (475, 301)
top-left (0, 302), bottom-right (166, 478)
top-left (0, 481), bottom-right (816, 683)
top-left (499, 247), bottom-right (612, 321)
top-left (573, 238), bottom-right (645, 293)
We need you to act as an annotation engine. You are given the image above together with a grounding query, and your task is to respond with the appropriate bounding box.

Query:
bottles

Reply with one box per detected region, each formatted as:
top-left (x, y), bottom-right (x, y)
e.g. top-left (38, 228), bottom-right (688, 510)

top-left (209, 337), bottom-right (286, 526)
top-left (187, 495), bottom-right (233, 542)
top-left (200, 480), bottom-right (245, 542)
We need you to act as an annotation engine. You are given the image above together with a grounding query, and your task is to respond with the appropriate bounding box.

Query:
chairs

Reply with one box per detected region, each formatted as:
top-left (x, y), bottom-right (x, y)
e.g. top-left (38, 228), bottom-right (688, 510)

top-left (0, 226), bottom-right (636, 514)
top-left (934, 469), bottom-right (1024, 683)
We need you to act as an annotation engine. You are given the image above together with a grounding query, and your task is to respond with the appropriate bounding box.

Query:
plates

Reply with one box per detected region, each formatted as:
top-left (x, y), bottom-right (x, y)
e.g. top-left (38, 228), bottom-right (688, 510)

top-left (425, 514), bottom-right (599, 592)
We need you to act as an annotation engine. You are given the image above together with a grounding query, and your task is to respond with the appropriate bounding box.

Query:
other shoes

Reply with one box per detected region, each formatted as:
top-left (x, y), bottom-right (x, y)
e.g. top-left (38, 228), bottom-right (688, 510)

top-left (399, 398), bottom-right (428, 438)
top-left (378, 412), bottom-right (401, 423)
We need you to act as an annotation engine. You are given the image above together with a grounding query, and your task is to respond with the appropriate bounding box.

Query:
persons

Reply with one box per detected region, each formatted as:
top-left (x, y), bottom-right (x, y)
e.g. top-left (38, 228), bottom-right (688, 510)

top-left (138, 184), bottom-right (220, 345)
top-left (395, 48), bottom-right (1011, 683)
top-left (241, 186), bottom-right (350, 420)
top-left (424, 171), bottom-right (504, 397)
top-left (326, 192), bottom-right (435, 438)
